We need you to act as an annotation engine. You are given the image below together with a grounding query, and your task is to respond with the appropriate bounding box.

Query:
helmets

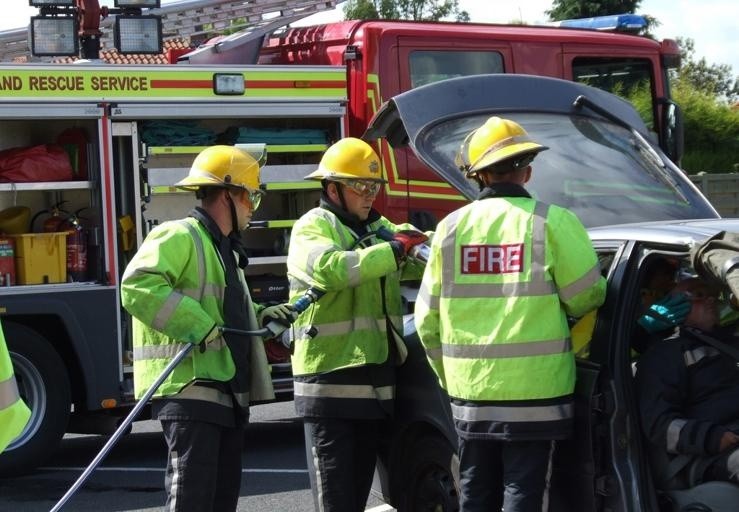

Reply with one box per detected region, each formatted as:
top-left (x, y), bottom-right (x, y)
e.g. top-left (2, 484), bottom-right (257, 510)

top-left (465, 115), bottom-right (550, 178)
top-left (174, 145), bottom-right (261, 194)
top-left (304, 137), bottom-right (389, 184)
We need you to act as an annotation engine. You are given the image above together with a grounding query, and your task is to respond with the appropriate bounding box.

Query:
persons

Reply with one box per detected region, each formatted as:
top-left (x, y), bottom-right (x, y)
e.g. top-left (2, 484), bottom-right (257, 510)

top-left (118, 144), bottom-right (299, 512)
top-left (414, 117), bottom-right (606, 512)
top-left (0, 314), bottom-right (33, 454)
top-left (635, 277), bottom-right (739, 491)
top-left (285, 136), bottom-right (434, 512)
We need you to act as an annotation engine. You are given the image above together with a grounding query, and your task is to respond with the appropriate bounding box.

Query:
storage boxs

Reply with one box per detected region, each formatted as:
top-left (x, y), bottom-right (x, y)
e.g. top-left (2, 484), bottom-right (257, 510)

top-left (3, 231), bottom-right (71, 286)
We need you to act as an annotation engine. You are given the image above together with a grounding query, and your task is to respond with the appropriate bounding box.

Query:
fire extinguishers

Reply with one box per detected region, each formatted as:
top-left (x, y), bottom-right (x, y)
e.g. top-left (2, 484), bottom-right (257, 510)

top-left (29, 199), bottom-right (90, 283)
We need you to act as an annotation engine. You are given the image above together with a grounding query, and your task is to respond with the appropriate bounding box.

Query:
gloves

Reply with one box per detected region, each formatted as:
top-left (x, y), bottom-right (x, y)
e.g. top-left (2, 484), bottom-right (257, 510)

top-left (637, 291), bottom-right (692, 335)
top-left (257, 303), bottom-right (296, 328)
top-left (389, 230), bottom-right (429, 260)
top-left (200, 325), bottom-right (219, 353)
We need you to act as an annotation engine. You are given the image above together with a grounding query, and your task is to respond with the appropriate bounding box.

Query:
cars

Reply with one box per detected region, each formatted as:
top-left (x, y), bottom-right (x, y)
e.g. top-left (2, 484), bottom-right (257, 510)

top-left (362, 76), bottom-right (739, 512)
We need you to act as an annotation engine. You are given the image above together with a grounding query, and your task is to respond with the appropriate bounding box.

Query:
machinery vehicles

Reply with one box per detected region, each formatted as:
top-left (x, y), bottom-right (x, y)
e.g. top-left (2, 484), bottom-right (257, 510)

top-left (0, 0), bottom-right (685, 479)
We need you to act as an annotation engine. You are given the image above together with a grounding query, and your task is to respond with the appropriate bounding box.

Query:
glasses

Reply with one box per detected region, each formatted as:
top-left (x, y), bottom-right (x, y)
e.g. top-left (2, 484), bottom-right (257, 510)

top-left (227, 188), bottom-right (262, 212)
top-left (326, 177), bottom-right (380, 196)
